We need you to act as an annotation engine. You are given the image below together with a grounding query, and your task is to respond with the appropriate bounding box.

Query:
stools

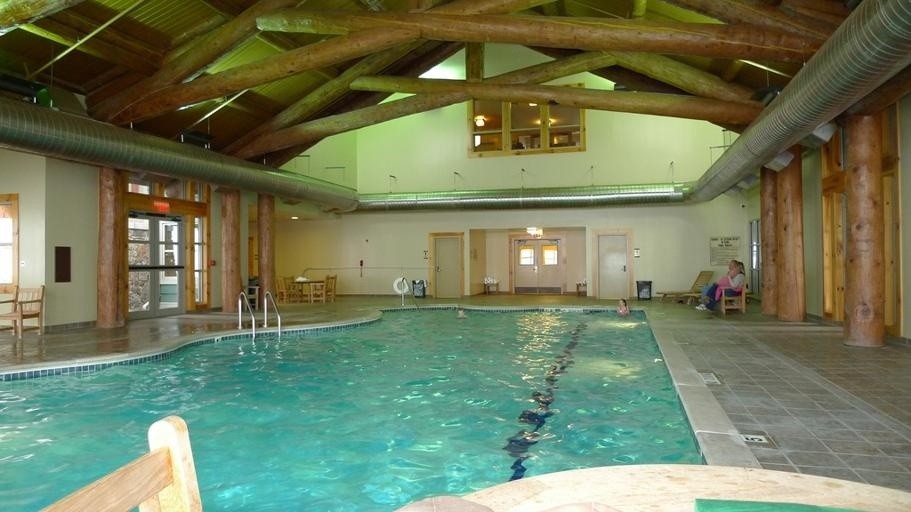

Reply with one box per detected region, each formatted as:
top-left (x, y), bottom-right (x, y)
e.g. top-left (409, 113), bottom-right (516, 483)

top-left (576, 283), bottom-right (587, 296)
top-left (483, 283), bottom-right (499, 296)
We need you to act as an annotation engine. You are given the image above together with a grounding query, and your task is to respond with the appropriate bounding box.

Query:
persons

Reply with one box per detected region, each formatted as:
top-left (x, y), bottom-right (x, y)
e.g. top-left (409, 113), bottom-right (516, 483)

top-left (695, 260), bottom-right (738, 311)
top-left (701, 261), bottom-right (746, 312)
top-left (617, 298), bottom-right (632, 317)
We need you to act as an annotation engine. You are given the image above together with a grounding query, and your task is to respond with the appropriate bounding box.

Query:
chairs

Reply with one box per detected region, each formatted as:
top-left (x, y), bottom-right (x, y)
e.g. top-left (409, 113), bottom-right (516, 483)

top-left (274, 274), bottom-right (337, 305)
top-left (684, 293), bottom-right (702, 305)
top-left (0, 284), bottom-right (45, 340)
top-left (656, 271), bottom-right (715, 305)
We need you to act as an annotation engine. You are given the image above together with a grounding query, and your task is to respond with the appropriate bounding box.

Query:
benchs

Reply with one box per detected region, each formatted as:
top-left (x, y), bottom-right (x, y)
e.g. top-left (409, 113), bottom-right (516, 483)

top-left (720, 279), bottom-right (746, 315)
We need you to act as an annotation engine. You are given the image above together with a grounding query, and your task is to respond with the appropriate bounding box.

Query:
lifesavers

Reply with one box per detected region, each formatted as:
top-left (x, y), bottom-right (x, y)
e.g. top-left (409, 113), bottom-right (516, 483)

top-left (393, 278), bottom-right (410, 294)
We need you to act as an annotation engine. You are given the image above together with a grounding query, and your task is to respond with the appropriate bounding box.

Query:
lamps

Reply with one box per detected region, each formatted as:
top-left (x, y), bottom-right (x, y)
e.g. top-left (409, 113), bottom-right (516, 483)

top-left (475, 115), bottom-right (486, 126)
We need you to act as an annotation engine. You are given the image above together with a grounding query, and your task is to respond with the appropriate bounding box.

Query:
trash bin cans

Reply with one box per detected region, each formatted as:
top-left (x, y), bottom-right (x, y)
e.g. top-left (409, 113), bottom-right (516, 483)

top-left (412, 279), bottom-right (427, 298)
top-left (636, 281), bottom-right (652, 300)
